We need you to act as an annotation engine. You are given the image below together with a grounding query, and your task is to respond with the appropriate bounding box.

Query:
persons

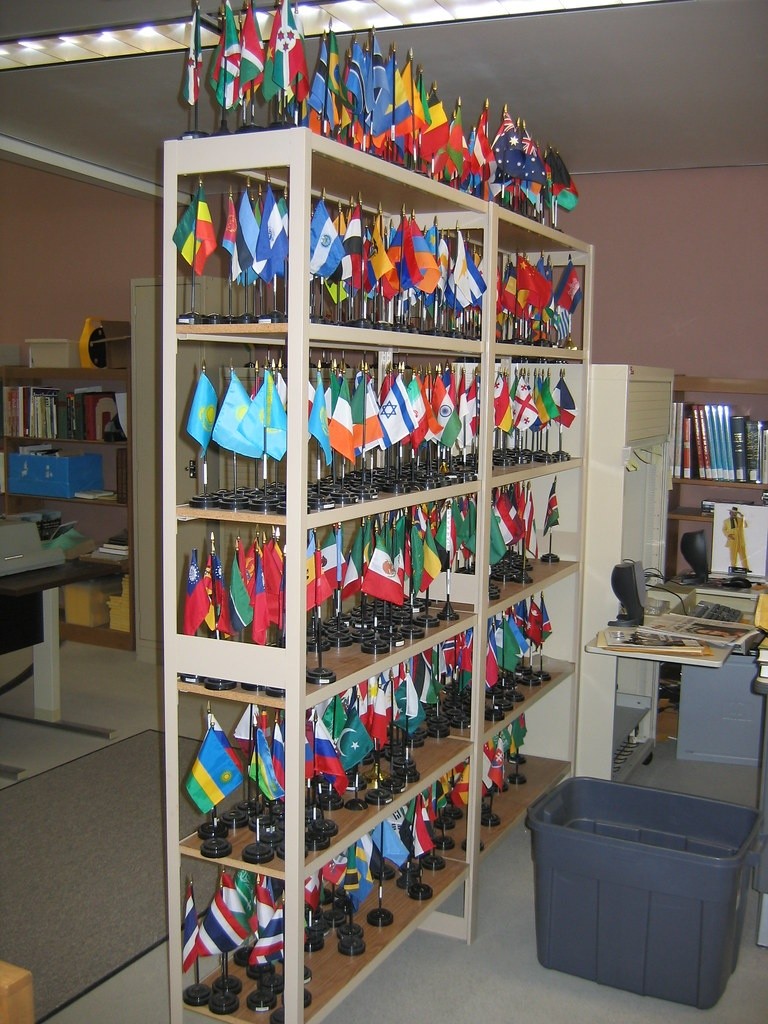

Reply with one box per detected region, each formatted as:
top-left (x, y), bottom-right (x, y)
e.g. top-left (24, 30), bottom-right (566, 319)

top-left (722, 507), bottom-right (752, 574)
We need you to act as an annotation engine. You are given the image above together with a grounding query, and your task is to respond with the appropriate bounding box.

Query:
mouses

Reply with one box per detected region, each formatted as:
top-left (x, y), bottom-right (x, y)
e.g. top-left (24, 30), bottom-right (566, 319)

top-left (721, 577), bottom-right (753, 588)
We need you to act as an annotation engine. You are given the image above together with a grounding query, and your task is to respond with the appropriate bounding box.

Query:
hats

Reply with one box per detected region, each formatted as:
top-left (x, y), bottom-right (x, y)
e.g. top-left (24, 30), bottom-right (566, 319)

top-left (728, 507), bottom-right (740, 513)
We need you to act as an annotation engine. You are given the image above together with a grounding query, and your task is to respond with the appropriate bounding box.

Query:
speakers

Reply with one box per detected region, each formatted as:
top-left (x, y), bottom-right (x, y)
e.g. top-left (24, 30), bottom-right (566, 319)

top-left (678, 530), bottom-right (709, 585)
top-left (608, 558), bottom-right (648, 627)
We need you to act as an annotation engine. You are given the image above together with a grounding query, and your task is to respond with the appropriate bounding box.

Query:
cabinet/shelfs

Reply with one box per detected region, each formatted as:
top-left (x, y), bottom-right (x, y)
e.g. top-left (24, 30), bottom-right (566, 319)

top-left (1, 364), bottom-right (137, 652)
top-left (645, 375), bottom-right (767, 596)
top-left (576, 365), bottom-right (677, 784)
top-left (156, 127), bottom-right (597, 1024)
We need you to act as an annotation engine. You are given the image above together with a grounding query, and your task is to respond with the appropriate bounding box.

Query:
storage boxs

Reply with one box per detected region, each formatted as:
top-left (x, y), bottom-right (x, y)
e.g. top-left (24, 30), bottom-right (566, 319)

top-left (6, 448), bottom-right (105, 500)
top-left (62, 575), bottom-right (123, 628)
top-left (523, 774), bottom-right (768, 1013)
top-left (24, 336), bottom-right (82, 369)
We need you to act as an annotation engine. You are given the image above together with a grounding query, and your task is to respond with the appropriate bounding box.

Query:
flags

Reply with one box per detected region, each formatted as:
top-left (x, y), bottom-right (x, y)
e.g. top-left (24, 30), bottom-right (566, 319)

top-left (155, 0), bottom-right (590, 1015)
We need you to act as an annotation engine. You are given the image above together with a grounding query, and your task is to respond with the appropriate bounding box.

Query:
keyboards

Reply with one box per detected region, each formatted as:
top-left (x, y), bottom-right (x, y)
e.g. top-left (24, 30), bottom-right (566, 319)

top-left (686, 601), bottom-right (743, 623)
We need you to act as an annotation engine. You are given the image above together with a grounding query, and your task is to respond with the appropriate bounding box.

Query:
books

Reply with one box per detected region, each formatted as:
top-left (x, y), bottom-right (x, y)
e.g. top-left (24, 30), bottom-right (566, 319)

top-left (0, 384), bottom-right (131, 639)
top-left (596, 613), bottom-right (755, 657)
top-left (669, 399), bottom-right (768, 519)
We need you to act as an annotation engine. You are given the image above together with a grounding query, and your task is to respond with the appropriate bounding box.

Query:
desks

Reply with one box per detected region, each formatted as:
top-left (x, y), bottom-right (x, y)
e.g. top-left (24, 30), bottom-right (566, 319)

top-left (0, 560), bottom-right (124, 782)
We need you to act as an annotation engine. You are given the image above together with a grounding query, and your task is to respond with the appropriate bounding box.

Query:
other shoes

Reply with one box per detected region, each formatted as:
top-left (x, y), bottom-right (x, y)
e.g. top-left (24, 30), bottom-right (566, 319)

top-left (743, 568), bottom-right (752, 572)
